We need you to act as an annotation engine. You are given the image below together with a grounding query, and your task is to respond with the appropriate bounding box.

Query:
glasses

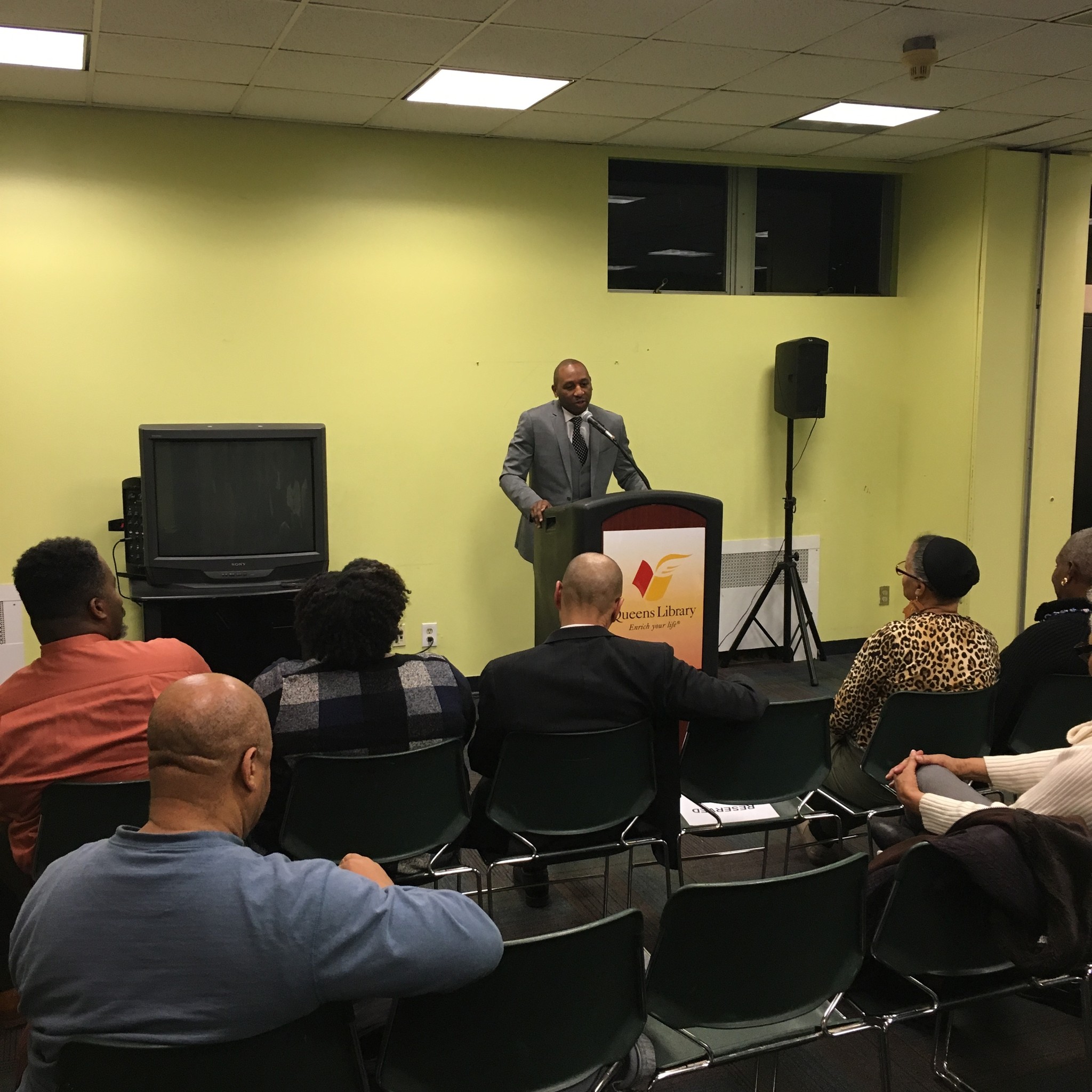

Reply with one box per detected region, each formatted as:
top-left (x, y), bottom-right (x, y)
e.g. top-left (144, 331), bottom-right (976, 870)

top-left (896, 561), bottom-right (932, 589)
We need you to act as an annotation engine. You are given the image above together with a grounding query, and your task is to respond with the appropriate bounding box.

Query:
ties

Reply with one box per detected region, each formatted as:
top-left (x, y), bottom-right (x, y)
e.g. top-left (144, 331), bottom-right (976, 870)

top-left (572, 417), bottom-right (588, 466)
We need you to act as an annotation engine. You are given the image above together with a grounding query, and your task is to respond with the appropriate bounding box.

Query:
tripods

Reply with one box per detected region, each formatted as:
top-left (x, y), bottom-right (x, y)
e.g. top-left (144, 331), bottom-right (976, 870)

top-left (721, 417), bottom-right (827, 687)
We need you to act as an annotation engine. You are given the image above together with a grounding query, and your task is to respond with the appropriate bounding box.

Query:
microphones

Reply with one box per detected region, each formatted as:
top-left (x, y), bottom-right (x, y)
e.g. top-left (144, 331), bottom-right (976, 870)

top-left (581, 411), bottom-right (617, 441)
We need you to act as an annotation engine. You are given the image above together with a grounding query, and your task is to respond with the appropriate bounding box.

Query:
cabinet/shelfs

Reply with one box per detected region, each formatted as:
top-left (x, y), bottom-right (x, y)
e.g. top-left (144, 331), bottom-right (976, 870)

top-left (129, 579), bottom-right (305, 685)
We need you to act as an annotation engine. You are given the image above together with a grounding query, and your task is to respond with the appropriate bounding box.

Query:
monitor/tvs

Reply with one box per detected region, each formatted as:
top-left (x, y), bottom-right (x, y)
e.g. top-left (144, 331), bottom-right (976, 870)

top-left (138, 423), bottom-right (330, 589)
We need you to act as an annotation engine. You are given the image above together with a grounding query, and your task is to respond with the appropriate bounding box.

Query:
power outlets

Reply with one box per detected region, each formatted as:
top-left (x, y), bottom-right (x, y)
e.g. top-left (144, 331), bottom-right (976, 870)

top-left (422, 623), bottom-right (437, 646)
top-left (880, 586), bottom-right (889, 605)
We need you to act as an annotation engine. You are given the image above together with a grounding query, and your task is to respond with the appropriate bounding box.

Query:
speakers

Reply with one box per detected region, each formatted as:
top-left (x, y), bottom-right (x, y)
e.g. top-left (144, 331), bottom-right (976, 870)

top-left (774, 337), bottom-right (828, 419)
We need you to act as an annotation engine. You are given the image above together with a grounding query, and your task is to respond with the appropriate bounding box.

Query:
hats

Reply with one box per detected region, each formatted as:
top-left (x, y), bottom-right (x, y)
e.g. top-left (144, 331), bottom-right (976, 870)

top-left (923, 537), bottom-right (979, 597)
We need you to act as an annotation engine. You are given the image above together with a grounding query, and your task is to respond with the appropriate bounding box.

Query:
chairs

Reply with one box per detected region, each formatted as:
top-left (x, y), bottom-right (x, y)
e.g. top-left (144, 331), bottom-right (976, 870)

top-left (35, 672), bottom-right (1092, 1092)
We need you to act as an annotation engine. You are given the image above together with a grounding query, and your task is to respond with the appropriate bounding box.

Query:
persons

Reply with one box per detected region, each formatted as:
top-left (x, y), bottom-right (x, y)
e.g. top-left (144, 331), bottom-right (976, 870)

top-left (460, 551), bottom-right (769, 907)
top-left (0, 537), bottom-right (214, 936)
top-left (796, 528), bottom-right (1092, 865)
top-left (250, 557), bottom-right (477, 883)
top-left (499, 359), bottom-right (651, 564)
top-left (8, 673), bottom-right (504, 1091)
